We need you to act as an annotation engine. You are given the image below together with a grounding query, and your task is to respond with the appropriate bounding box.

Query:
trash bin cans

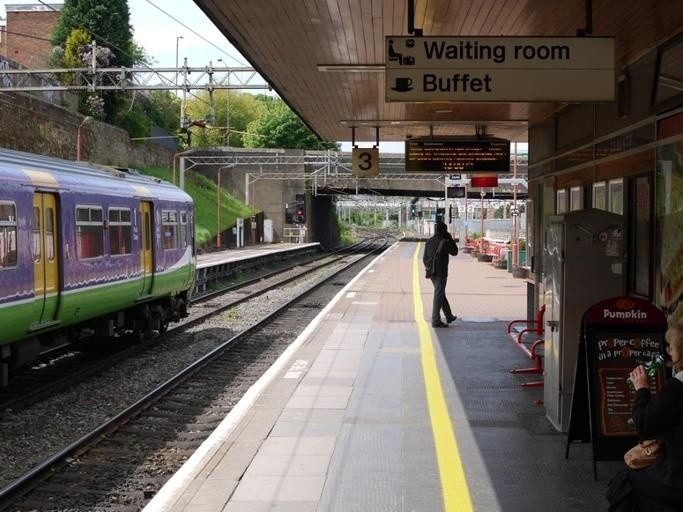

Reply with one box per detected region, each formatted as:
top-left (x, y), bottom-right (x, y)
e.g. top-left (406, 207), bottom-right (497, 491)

top-left (507, 250), bottom-right (527, 272)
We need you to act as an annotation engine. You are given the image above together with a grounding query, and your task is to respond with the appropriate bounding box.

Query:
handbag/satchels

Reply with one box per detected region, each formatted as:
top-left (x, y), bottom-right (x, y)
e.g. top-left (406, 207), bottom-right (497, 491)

top-left (624, 437), bottom-right (663, 470)
top-left (425, 258), bottom-right (437, 278)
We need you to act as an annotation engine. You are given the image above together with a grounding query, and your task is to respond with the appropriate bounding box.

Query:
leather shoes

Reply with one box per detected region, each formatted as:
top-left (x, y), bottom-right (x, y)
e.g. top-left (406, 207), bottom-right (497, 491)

top-left (447, 316), bottom-right (457, 324)
top-left (432, 322), bottom-right (448, 328)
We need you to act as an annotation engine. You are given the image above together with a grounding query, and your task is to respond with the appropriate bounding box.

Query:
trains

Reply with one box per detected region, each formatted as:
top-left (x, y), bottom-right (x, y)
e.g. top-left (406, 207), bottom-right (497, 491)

top-left (0, 146), bottom-right (200, 390)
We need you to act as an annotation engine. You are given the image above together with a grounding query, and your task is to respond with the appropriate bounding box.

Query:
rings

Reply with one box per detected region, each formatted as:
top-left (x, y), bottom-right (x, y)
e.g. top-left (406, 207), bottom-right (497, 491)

top-left (634, 373), bottom-right (637, 376)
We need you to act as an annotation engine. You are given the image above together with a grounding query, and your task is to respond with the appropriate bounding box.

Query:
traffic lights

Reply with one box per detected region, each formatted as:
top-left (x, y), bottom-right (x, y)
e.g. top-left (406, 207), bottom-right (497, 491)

top-left (295, 192), bottom-right (307, 224)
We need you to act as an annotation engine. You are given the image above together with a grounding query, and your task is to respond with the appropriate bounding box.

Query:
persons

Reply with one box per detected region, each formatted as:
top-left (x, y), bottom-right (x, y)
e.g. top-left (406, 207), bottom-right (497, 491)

top-left (422, 221), bottom-right (457, 328)
top-left (605, 318), bottom-right (683, 512)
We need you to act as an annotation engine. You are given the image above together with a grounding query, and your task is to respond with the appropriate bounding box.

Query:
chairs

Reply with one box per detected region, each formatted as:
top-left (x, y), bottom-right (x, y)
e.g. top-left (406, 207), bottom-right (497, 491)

top-left (507, 304), bottom-right (544, 404)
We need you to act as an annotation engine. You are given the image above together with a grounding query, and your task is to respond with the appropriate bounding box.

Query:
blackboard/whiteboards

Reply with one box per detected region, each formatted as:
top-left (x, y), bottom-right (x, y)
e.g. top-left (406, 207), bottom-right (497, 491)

top-left (583, 296), bottom-right (667, 437)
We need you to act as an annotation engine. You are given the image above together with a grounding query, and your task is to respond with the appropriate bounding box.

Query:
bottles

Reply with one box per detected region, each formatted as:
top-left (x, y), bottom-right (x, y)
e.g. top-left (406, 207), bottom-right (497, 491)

top-left (626, 353), bottom-right (666, 391)
